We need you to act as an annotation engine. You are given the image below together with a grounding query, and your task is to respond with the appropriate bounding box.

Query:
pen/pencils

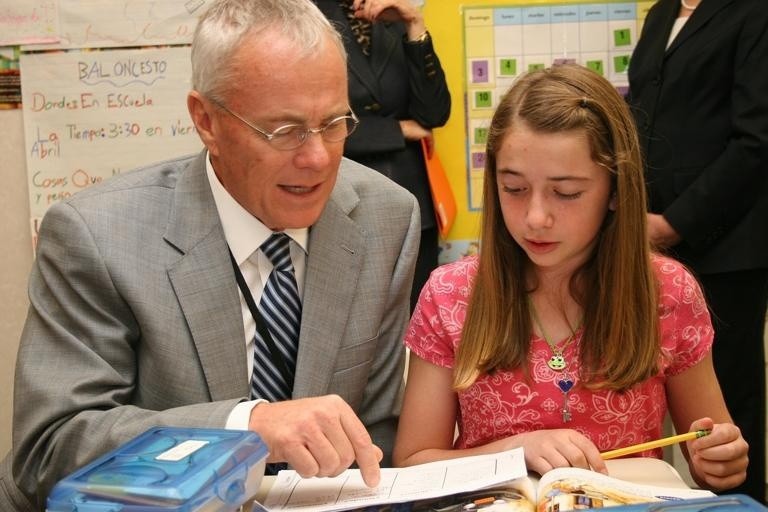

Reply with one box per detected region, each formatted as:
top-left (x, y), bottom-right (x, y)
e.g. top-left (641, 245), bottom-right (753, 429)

top-left (600, 428), bottom-right (711, 461)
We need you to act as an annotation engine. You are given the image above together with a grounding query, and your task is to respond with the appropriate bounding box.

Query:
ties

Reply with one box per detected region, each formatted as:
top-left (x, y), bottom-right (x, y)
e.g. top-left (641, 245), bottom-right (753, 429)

top-left (249, 229), bottom-right (304, 474)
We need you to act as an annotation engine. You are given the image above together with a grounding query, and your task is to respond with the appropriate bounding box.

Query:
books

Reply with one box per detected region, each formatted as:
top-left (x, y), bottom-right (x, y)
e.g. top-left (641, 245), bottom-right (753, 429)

top-left (358, 467), bottom-right (718, 511)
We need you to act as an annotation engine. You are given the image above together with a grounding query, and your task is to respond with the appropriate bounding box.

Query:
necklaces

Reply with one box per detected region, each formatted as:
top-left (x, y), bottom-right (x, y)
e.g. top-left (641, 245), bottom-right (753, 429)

top-left (521, 284), bottom-right (588, 372)
top-left (681, 0), bottom-right (698, 11)
top-left (532, 296), bottom-right (585, 423)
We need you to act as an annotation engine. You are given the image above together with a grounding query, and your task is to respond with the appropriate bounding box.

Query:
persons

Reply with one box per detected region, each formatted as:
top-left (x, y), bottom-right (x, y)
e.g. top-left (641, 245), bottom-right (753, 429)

top-left (0, 0), bottom-right (423, 512)
top-left (393, 60), bottom-right (749, 489)
top-left (622, 1), bottom-right (767, 504)
top-left (312, 1), bottom-right (452, 319)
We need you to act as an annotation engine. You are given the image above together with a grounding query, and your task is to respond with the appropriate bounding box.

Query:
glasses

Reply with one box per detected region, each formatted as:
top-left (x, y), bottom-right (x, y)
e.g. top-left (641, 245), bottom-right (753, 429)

top-left (202, 90), bottom-right (361, 153)
